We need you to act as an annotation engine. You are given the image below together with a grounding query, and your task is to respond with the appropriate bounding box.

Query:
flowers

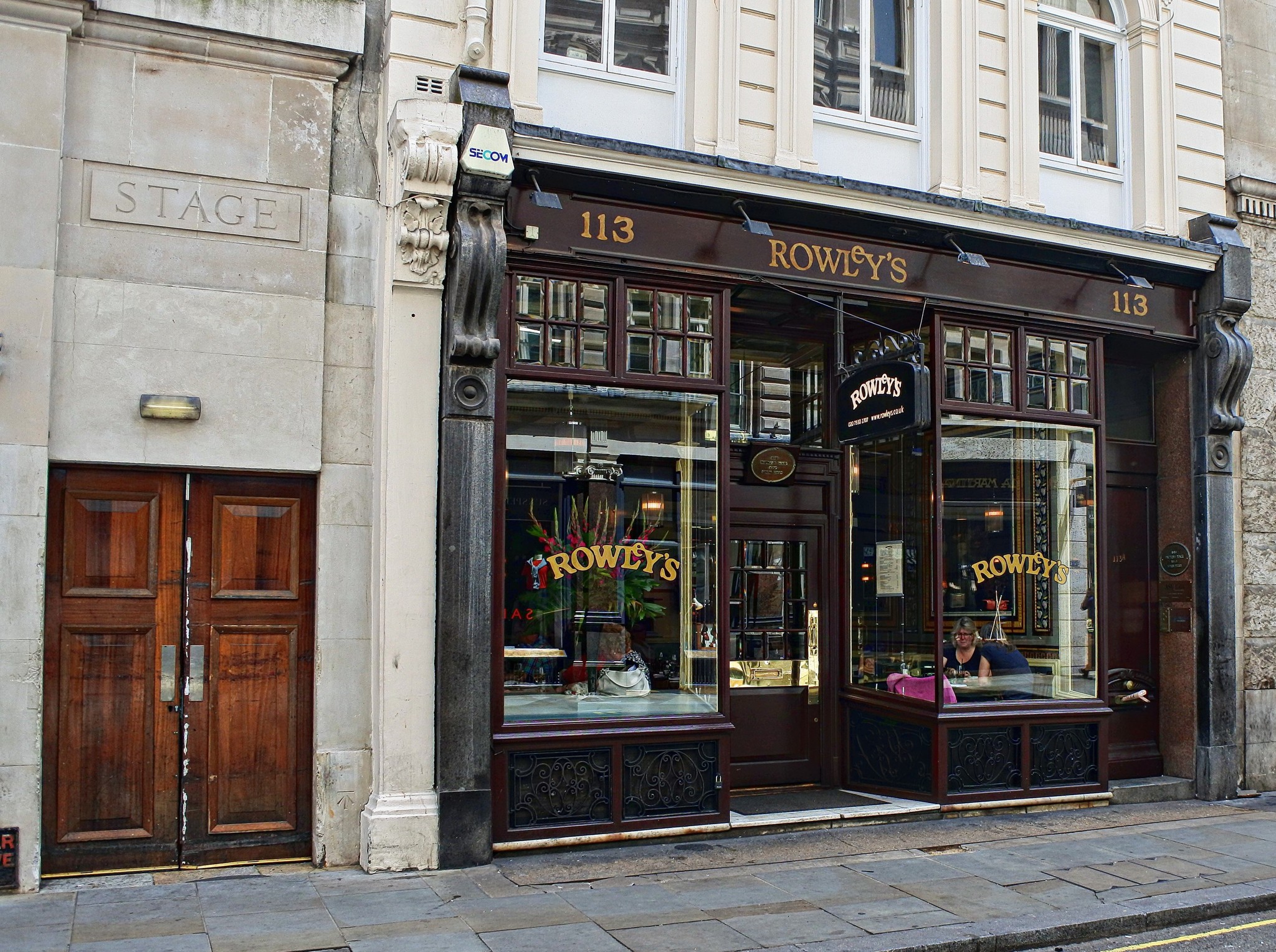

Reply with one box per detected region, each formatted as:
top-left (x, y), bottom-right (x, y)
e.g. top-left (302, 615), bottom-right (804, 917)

top-left (519, 493), bottom-right (668, 633)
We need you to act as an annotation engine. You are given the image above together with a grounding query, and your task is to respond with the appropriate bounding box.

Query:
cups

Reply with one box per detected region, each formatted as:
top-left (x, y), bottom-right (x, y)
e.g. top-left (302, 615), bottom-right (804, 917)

top-left (956, 665), bottom-right (965, 679)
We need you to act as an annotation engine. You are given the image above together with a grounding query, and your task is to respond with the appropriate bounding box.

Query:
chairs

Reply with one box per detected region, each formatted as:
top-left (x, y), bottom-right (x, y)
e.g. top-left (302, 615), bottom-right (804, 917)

top-left (885, 672), bottom-right (958, 705)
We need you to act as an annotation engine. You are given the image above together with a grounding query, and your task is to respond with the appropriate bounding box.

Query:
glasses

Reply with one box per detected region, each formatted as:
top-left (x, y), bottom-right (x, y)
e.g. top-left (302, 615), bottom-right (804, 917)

top-left (956, 632), bottom-right (973, 638)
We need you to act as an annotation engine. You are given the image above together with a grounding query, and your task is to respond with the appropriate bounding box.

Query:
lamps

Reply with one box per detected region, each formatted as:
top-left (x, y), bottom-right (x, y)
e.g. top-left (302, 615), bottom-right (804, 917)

top-left (984, 506), bottom-right (1004, 533)
top-left (942, 233), bottom-right (990, 268)
top-left (692, 598), bottom-right (703, 610)
top-left (140, 394), bottom-right (201, 420)
top-left (1104, 259), bottom-right (1154, 290)
top-left (731, 199), bottom-right (774, 237)
top-left (526, 167), bottom-right (563, 209)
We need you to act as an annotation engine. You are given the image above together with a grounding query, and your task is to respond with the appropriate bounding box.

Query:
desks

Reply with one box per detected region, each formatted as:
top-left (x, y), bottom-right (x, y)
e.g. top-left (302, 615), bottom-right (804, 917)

top-left (947, 673), bottom-right (981, 694)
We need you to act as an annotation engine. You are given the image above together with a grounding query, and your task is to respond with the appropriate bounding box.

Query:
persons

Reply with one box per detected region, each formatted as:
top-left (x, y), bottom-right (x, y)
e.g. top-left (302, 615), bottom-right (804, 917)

top-left (1079, 581), bottom-right (1094, 678)
top-left (504, 613), bottom-right (650, 696)
top-left (942, 616), bottom-right (1034, 702)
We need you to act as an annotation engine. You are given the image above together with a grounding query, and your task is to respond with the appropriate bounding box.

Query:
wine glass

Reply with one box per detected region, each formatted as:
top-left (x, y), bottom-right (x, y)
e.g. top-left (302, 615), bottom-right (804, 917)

top-left (513, 663), bottom-right (524, 691)
top-left (532, 667), bottom-right (544, 694)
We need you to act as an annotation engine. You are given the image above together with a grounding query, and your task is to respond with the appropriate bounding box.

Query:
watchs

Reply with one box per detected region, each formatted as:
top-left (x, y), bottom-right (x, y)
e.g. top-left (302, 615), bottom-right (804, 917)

top-left (566, 684), bottom-right (570, 690)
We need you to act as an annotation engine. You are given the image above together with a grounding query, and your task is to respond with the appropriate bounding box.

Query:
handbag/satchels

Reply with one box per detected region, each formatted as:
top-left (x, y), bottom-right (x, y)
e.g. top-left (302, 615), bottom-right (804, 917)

top-left (596, 667), bottom-right (650, 697)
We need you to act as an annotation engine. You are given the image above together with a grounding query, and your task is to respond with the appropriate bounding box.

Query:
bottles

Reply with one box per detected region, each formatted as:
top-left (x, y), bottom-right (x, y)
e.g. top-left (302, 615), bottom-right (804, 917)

top-left (898, 650), bottom-right (908, 675)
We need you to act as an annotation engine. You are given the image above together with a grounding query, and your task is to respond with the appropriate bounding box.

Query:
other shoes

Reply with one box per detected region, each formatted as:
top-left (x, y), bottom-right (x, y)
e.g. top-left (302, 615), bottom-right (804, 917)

top-left (1079, 668), bottom-right (1093, 676)
